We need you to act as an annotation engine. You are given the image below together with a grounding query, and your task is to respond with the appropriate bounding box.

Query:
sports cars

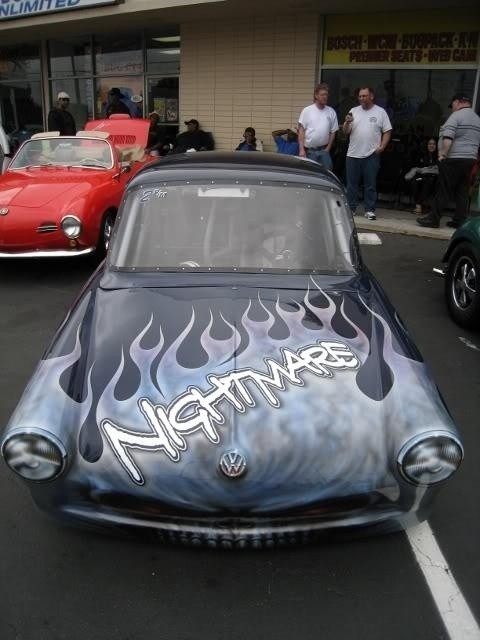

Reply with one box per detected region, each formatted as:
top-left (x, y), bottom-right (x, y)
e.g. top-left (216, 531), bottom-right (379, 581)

top-left (0, 109), bottom-right (163, 269)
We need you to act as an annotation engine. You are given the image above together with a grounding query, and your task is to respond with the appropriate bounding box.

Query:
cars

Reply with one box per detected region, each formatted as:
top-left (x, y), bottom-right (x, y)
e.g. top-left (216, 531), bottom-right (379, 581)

top-left (1, 144), bottom-right (467, 555)
top-left (433, 179), bottom-right (479, 325)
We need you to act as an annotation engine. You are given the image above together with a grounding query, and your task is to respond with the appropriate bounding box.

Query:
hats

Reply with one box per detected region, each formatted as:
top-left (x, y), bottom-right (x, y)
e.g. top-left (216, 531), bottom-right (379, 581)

top-left (287, 127), bottom-right (297, 136)
top-left (111, 88), bottom-right (124, 99)
top-left (448, 92), bottom-right (472, 108)
top-left (185, 120), bottom-right (199, 127)
top-left (58, 92), bottom-right (72, 101)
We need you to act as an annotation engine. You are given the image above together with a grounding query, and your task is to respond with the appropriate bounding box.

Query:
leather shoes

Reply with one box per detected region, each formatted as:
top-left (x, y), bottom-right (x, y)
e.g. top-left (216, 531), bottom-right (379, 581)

top-left (417, 217), bottom-right (439, 228)
top-left (446, 220), bottom-right (462, 227)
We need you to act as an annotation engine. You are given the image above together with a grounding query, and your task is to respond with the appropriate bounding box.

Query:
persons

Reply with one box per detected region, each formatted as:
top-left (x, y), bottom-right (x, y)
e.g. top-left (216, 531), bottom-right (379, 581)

top-left (105, 87), bottom-right (133, 120)
top-left (410, 135), bottom-right (442, 215)
top-left (298, 83), bottom-right (339, 175)
top-left (144, 112), bottom-right (170, 157)
top-left (271, 127), bottom-right (300, 156)
top-left (171, 119), bottom-right (215, 155)
top-left (235, 127), bottom-right (264, 152)
top-left (342, 86), bottom-right (393, 220)
top-left (48, 91), bottom-right (80, 150)
top-left (416, 93), bottom-right (480, 228)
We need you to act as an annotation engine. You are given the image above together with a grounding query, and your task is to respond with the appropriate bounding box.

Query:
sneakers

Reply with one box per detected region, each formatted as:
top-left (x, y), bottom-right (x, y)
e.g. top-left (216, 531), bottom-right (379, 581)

top-left (364, 211), bottom-right (376, 220)
